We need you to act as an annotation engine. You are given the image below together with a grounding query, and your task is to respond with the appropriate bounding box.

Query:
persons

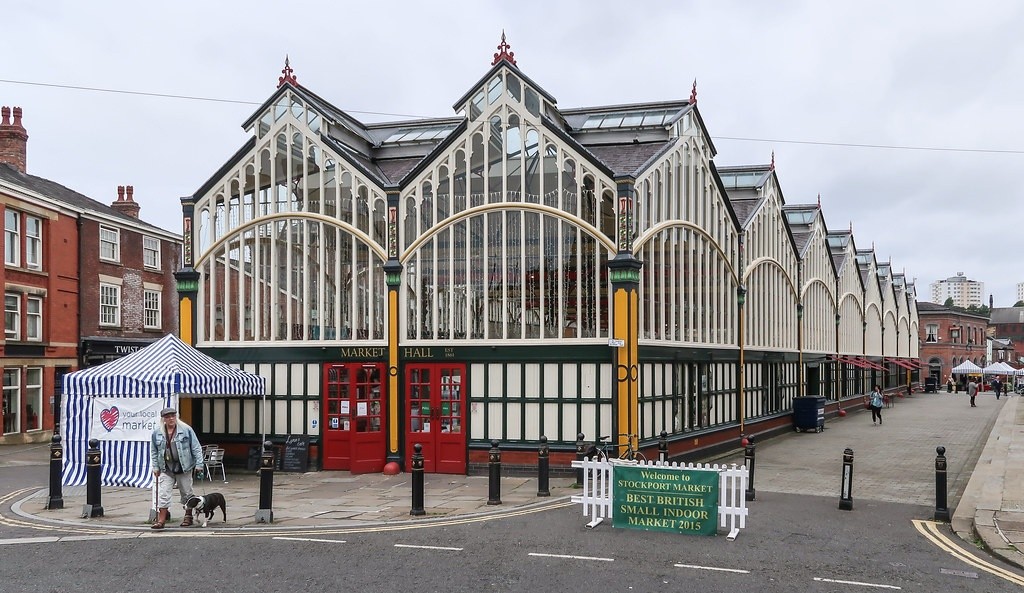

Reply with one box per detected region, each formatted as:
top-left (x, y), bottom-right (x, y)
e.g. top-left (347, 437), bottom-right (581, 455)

top-left (151, 408), bottom-right (204, 530)
top-left (933, 374), bottom-right (938, 394)
top-left (992, 375), bottom-right (1003, 399)
top-left (969, 378), bottom-right (978, 407)
top-left (870, 385), bottom-right (883, 425)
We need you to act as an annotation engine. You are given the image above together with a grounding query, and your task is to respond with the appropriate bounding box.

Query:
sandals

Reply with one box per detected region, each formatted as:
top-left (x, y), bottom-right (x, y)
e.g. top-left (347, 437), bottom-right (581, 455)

top-left (151, 522), bottom-right (165, 529)
top-left (180, 514), bottom-right (193, 526)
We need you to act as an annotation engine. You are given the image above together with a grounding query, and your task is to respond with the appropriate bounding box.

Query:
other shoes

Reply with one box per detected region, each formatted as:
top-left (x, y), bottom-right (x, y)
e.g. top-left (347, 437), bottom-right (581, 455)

top-left (971, 405), bottom-right (976, 407)
top-left (880, 417), bottom-right (882, 424)
top-left (874, 422), bottom-right (876, 426)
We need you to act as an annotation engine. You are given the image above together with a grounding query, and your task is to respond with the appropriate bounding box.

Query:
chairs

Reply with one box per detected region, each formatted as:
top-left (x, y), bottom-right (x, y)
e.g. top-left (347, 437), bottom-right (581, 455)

top-left (194, 444), bottom-right (218, 478)
top-left (194, 449), bottom-right (226, 482)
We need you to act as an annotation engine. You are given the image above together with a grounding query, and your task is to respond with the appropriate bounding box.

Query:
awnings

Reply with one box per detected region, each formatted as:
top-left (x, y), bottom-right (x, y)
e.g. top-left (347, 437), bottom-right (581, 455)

top-left (901, 359), bottom-right (922, 369)
top-left (830, 355), bottom-right (889, 371)
top-left (929, 363), bottom-right (941, 366)
top-left (886, 357), bottom-right (912, 369)
top-left (912, 359), bottom-right (928, 365)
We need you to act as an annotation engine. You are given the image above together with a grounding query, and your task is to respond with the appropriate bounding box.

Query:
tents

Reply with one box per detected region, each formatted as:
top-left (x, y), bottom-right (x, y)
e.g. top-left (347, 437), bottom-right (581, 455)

top-left (1012, 368), bottom-right (1024, 393)
top-left (60, 333), bottom-right (266, 489)
top-left (983, 362), bottom-right (1008, 392)
top-left (1000, 362), bottom-right (1017, 393)
top-left (951, 360), bottom-right (983, 393)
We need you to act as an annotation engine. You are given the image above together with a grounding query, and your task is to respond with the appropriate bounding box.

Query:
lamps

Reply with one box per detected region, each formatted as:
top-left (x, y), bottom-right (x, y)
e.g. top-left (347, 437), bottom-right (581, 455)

top-left (938, 336), bottom-right (942, 340)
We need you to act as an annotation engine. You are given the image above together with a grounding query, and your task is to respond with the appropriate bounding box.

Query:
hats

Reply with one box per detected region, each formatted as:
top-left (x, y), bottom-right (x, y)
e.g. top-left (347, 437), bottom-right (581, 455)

top-left (160, 408), bottom-right (177, 417)
top-left (996, 376), bottom-right (1000, 379)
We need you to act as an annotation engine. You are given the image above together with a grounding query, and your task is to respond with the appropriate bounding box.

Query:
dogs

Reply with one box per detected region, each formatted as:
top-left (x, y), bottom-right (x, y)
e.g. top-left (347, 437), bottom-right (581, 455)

top-left (183, 493), bottom-right (226, 528)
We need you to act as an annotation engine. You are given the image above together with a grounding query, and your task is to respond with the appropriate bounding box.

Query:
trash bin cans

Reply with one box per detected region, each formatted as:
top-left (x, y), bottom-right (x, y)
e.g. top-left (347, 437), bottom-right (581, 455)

top-left (792, 395), bottom-right (826, 433)
top-left (925, 377), bottom-right (937, 393)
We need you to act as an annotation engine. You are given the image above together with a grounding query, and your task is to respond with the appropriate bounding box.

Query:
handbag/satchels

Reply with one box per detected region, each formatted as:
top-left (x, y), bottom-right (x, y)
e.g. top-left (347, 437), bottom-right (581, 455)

top-left (867, 394), bottom-right (872, 410)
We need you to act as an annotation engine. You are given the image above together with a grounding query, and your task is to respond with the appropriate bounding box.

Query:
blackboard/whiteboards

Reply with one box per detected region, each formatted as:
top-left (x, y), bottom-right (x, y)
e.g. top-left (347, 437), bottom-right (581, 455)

top-left (282, 434), bottom-right (309, 472)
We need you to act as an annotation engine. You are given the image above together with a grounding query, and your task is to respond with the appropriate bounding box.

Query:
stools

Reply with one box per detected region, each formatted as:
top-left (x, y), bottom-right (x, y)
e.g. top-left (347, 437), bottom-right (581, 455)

top-left (885, 393), bottom-right (894, 408)
